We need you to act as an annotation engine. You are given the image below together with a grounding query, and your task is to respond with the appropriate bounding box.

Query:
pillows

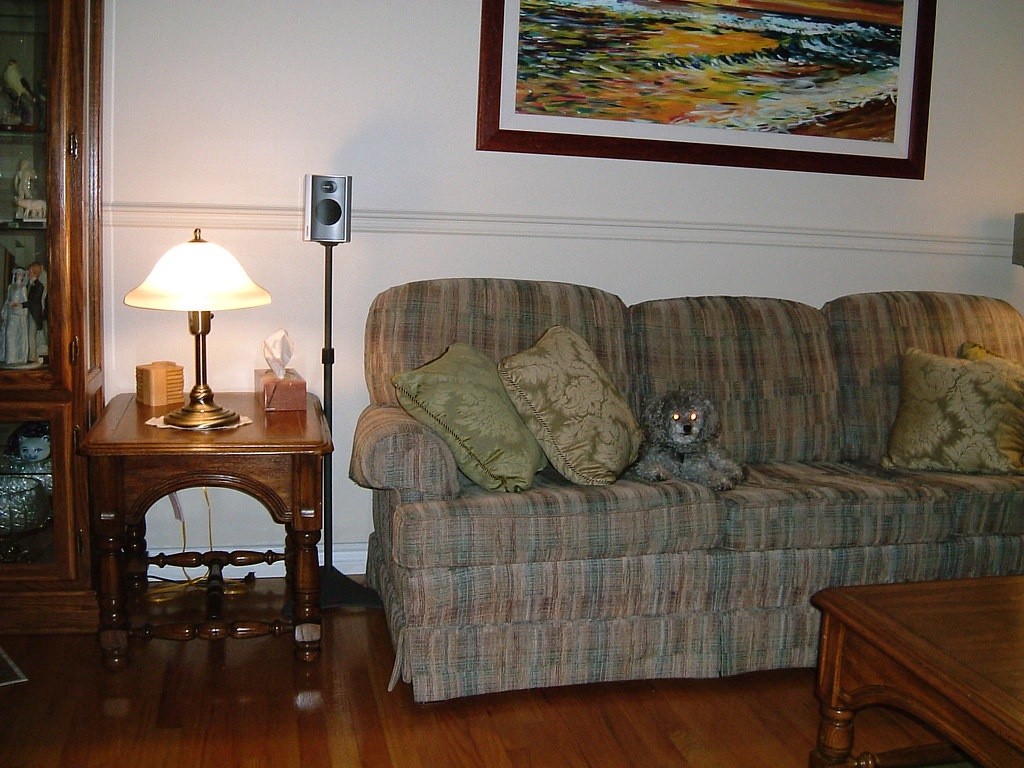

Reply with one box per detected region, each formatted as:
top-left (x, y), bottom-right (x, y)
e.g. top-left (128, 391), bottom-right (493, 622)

top-left (390, 340), bottom-right (547, 493)
top-left (497, 324), bottom-right (648, 488)
top-left (880, 339), bottom-right (1024, 477)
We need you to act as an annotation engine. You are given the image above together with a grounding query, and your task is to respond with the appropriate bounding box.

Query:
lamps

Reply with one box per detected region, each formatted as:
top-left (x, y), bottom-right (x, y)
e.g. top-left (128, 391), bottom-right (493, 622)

top-left (122, 227), bottom-right (274, 432)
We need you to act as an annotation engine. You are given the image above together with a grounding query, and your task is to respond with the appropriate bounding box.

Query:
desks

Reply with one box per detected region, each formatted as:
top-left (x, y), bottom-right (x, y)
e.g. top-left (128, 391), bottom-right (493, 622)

top-left (78, 391), bottom-right (336, 670)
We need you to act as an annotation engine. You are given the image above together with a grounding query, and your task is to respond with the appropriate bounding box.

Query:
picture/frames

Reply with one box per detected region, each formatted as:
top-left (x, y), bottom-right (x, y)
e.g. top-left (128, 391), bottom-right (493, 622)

top-left (475, 0), bottom-right (936, 181)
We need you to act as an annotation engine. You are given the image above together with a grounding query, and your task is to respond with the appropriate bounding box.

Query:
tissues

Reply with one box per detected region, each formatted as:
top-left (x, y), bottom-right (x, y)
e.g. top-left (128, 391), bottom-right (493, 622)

top-left (255, 329), bottom-right (306, 412)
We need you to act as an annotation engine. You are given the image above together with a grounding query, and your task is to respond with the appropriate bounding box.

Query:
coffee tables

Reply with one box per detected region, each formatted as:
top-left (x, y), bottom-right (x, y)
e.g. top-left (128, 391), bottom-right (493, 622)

top-left (809, 576), bottom-right (1024, 768)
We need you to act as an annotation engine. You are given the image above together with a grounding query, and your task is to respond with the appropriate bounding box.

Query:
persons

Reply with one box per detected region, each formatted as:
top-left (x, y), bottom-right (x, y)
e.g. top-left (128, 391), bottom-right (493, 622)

top-left (0, 262), bottom-right (44, 366)
top-left (14, 160), bottom-right (38, 219)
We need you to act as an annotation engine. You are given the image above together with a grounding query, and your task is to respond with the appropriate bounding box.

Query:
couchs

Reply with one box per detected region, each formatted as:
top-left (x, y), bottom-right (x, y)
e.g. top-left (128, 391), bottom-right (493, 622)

top-left (347, 278), bottom-right (1024, 704)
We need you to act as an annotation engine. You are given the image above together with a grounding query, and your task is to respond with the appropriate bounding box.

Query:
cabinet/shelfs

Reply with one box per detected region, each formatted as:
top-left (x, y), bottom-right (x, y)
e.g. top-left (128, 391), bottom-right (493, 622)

top-left (0, 0), bottom-right (107, 639)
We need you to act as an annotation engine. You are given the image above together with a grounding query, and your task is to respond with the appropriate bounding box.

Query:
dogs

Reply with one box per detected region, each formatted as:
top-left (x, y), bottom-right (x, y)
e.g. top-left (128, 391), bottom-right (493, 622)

top-left (635, 391), bottom-right (745, 492)
